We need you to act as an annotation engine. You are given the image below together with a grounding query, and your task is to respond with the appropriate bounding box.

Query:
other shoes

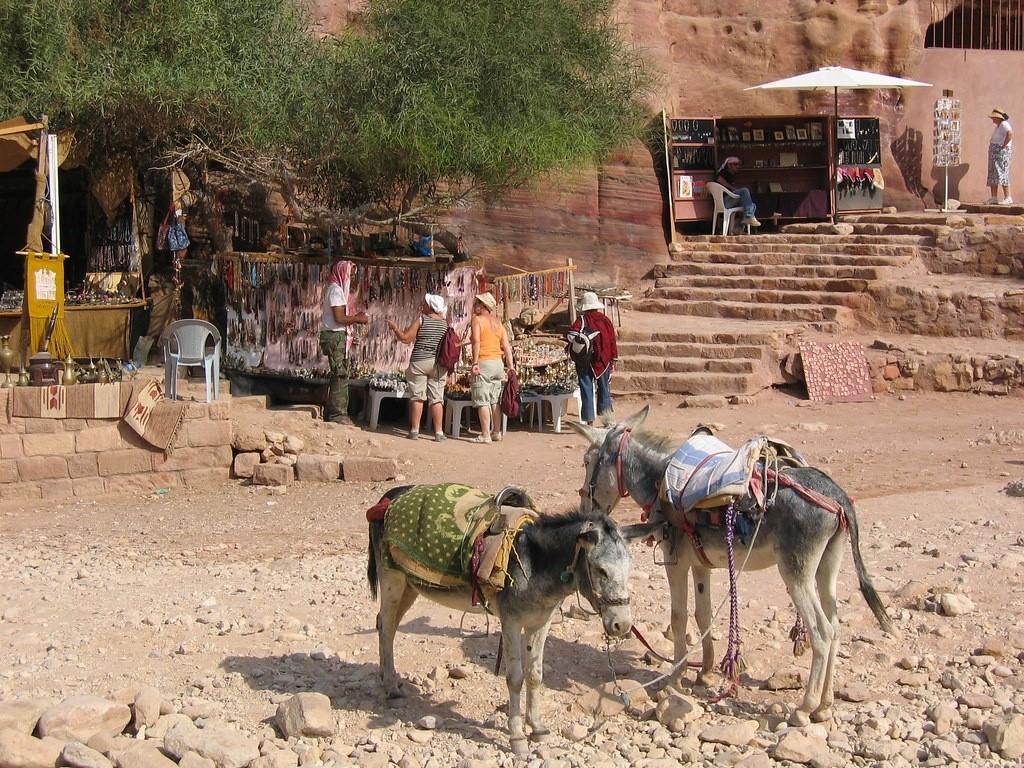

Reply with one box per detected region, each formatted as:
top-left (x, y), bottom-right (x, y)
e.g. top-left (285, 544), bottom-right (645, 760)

top-left (327, 415), bottom-right (356, 424)
top-left (407, 431), bottom-right (418, 440)
top-left (490, 431), bottom-right (502, 441)
top-left (743, 216), bottom-right (761, 226)
top-left (468, 436), bottom-right (492, 444)
top-left (983, 198), bottom-right (998, 205)
top-left (434, 433), bottom-right (447, 441)
top-left (732, 225), bottom-right (748, 235)
top-left (998, 197), bottom-right (1013, 204)
top-left (148, 358), bottom-right (166, 365)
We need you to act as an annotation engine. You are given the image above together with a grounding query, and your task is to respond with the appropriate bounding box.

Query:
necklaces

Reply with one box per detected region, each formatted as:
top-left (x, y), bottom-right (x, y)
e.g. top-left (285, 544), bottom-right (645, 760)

top-left (679, 120), bottom-right (683, 132)
top-left (856, 119), bottom-right (878, 139)
top-left (672, 120), bottom-right (677, 132)
top-left (684, 120), bottom-right (689, 132)
top-left (839, 139), bottom-right (880, 164)
top-left (693, 120), bottom-right (699, 130)
top-left (674, 147), bottom-right (713, 167)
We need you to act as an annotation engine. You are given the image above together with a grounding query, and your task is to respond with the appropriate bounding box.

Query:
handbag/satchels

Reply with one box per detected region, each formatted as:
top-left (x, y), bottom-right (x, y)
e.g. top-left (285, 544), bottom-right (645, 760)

top-left (156, 205), bottom-right (191, 250)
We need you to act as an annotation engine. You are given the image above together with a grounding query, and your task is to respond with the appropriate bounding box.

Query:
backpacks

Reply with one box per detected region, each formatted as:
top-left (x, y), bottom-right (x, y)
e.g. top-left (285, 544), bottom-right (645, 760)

top-left (565, 316), bottom-right (600, 367)
top-left (500, 370), bottom-right (522, 416)
top-left (436, 319), bottom-right (462, 367)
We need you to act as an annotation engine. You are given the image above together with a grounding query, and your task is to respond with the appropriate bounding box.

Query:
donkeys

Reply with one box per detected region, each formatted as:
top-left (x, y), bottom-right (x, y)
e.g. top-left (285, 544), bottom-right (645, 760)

top-left (365, 481), bottom-right (669, 758)
top-left (558, 400), bottom-right (902, 726)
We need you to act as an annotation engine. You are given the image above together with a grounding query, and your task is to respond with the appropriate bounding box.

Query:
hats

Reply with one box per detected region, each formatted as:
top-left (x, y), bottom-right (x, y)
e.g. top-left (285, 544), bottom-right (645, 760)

top-left (474, 292), bottom-right (496, 312)
top-left (987, 108), bottom-right (1006, 120)
top-left (727, 157), bottom-right (739, 164)
top-left (424, 293), bottom-right (445, 315)
top-left (575, 292), bottom-right (605, 311)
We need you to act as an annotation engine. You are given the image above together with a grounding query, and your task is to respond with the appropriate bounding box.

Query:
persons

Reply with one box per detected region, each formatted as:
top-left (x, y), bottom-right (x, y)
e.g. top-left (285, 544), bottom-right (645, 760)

top-left (788, 128), bottom-right (796, 140)
top-left (567, 292), bottom-right (618, 427)
top-left (717, 157), bottom-right (761, 235)
top-left (986, 108), bottom-right (1013, 205)
top-left (469, 292), bottom-right (516, 444)
top-left (318, 260), bottom-right (368, 426)
top-left (384, 293), bottom-right (449, 441)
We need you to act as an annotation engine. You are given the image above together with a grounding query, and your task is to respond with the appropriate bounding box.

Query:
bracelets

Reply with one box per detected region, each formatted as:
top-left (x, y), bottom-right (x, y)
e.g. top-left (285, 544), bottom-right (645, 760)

top-left (473, 363), bottom-right (478, 365)
top-left (394, 328), bottom-right (398, 331)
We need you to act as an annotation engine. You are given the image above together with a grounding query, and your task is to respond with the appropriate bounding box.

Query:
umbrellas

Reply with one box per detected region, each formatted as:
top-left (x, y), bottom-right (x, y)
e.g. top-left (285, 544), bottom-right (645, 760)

top-left (743, 66), bottom-right (934, 114)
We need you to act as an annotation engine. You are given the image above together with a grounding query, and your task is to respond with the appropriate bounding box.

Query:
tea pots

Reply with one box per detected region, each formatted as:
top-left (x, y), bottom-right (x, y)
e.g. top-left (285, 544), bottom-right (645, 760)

top-left (56, 349), bottom-right (80, 386)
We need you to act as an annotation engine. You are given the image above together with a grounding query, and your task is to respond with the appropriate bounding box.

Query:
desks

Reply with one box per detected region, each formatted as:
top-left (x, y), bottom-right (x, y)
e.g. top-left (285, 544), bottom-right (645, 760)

top-left (552, 293), bottom-right (634, 328)
top-left (0, 300), bottom-right (148, 365)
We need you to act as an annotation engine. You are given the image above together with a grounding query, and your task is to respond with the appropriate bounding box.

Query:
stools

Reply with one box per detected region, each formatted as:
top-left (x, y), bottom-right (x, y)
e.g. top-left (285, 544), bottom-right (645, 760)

top-left (520, 397), bottom-right (543, 434)
top-left (446, 398), bottom-right (508, 439)
top-left (366, 388), bottom-right (433, 433)
top-left (529, 388), bottom-right (586, 432)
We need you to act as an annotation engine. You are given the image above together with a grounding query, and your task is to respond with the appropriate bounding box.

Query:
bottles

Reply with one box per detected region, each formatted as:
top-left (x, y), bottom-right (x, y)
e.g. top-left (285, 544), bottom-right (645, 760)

top-left (81, 352), bottom-right (108, 384)
top-left (0, 335), bottom-right (29, 387)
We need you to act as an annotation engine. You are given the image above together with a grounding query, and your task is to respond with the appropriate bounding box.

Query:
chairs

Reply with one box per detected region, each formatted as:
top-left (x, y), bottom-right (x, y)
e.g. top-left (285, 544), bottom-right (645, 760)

top-left (706, 181), bottom-right (751, 236)
top-left (159, 318), bottom-right (222, 404)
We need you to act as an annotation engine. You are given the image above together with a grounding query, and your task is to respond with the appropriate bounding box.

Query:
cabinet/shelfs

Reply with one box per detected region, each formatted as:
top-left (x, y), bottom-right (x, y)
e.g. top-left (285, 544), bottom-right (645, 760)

top-left (669, 112), bottom-right (836, 226)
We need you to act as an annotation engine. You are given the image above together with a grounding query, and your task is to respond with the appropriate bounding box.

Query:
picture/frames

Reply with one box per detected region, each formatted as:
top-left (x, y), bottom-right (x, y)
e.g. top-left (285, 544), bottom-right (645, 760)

top-left (752, 129), bottom-right (764, 142)
top-left (796, 128), bottom-right (808, 140)
top-left (785, 124), bottom-right (797, 140)
top-left (742, 131), bottom-right (751, 141)
top-left (773, 131), bottom-right (785, 141)
